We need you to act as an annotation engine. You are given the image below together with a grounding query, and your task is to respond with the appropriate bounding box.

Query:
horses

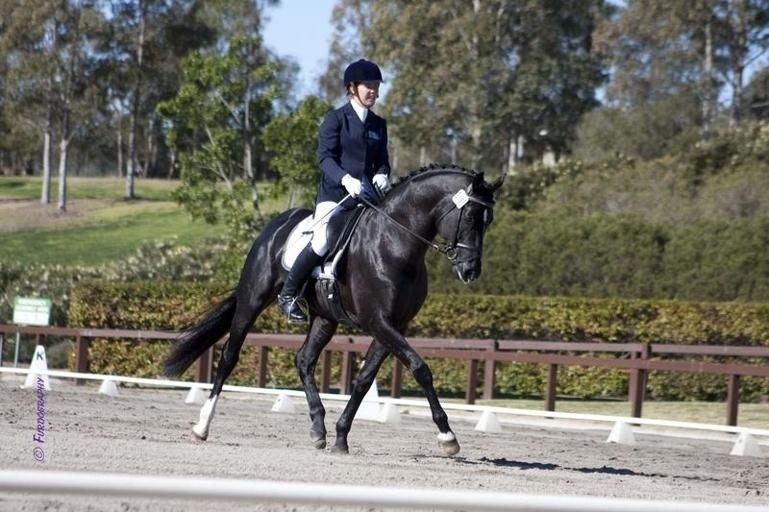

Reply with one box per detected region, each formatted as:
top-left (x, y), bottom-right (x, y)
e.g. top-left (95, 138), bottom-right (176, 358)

top-left (159, 162), bottom-right (507, 456)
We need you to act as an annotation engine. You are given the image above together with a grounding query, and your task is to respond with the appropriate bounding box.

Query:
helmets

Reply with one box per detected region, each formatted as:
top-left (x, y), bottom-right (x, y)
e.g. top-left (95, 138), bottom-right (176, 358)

top-left (344, 59), bottom-right (382, 86)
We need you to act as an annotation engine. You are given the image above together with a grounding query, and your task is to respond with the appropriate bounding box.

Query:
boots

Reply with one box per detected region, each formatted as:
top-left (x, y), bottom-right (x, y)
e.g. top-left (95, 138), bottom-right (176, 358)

top-left (277, 241), bottom-right (329, 322)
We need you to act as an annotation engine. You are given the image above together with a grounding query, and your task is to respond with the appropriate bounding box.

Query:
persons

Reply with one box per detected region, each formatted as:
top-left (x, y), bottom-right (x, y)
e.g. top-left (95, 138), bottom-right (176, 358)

top-left (278, 59), bottom-right (394, 323)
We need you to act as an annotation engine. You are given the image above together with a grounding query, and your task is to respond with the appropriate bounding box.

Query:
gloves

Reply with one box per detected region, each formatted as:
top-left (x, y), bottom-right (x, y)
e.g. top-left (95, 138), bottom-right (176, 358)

top-left (372, 173), bottom-right (393, 193)
top-left (341, 173), bottom-right (365, 198)
